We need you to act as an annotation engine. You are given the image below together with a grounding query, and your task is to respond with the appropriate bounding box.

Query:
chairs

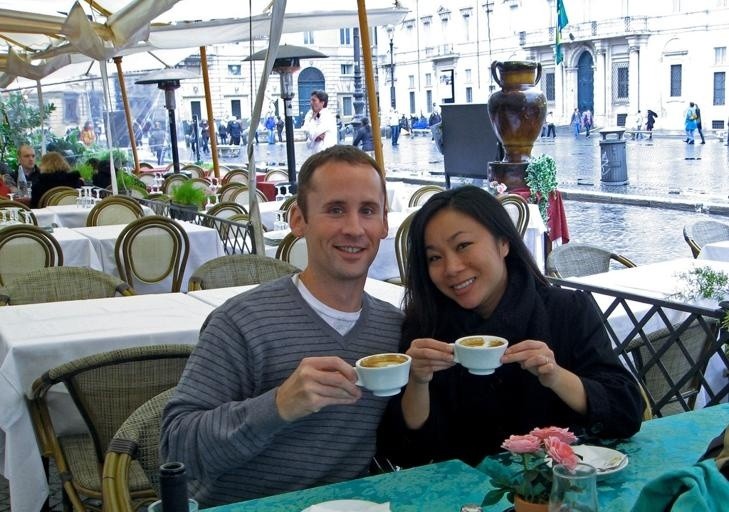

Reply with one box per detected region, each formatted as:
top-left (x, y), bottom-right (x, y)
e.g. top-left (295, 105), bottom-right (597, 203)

top-left (39, 186), bottom-right (71, 206)
top-left (263, 171), bottom-right (289, 181)
top-left (181, 165), bottom-right (205, 177)
top-left (1, 265), bottom-right (137, 304)
top-left (0, 223), bottom-right (65, 281)
top-left (682, 216), bottom-right (729, 252)
top-left (113, 214), bottom-right (190, 293)
top-left (1, 195), bottom-right (38, 230)
top-left (139, 161), bottom-right (153, 170)
top-left (28, 344), bottom-right (196, 512)
top-left (208, 164), bottom-right (229, 178)
top-left (48, 189), bottom-right (87, 207)
top-left (202, 202), bottom-right (250, 229)
top-left (546, 242), bottom-right (639, 278)
top-left (165, 163), bottom-right (184, 170)
top-left (222, 215), bottom-right (268, 241)
top-left (496, 193), bottom-right (530, 235)
top-left (218, 181), bottom-right (244, 202)
top-left (221, 169), bottom-right (250, 184)
top-left (103, 383), bottom-right (185, 512)
top-left (188, 254), bottom-right (300, 289)
top-left (140, 162), bottom-right (154, 169)
top-left (408, 183), bottom-right (442, 209)
top-left (386, 209), bottom-right (418, 289)
top-left (280, 198), bottom-right (298, 222)
top-left (162, 173), bottom-right (188, 196)
top-left (619, 315), bottom-right (722, 421)
top-left (277, 231), bottom-right (310, 272)
top-left (86, 197), bottom-right (144, 225)
top-left (137, 173), bottom-right (155, 186)
top-left (190, 178), bottom-right (212, 187)
top-left (229, 187), bottom-right (268, 208)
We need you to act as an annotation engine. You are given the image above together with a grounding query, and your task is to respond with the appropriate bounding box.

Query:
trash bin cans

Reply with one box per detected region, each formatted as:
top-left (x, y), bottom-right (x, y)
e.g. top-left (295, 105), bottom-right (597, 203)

top-left (598, 126), bottom-right (630, 186)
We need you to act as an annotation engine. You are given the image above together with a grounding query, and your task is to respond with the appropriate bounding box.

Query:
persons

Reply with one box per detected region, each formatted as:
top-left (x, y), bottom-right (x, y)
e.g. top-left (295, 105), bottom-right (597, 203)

top-left (352, 117), bottom-right (375, 159)
top-left (569, 108), bottom-right (582, 138)
top-left (132, 116), bottom-right (243, 165)
top-left (265, 117), bottom-right (276, 144)
top-left (301, 90), bottom-right (338, 154)
top-left (545, 111), bottom-right (557, 139)
top-left (159, 143), bottom-right (407, 509)
top-left (336, 114), bottom-right (345, 142)
top-left (583, 110), bottom-right (592, 139)
top-left (388, 107), bottom-right (441, 146)
top-left (277, 116), bottom-right (284, 143)
top-left (636, 109), bottom-right (643, 139)
top-left (685, 102), bottom-right (697, 144)
top-left (645, 109), bottom-right (658, 139)
top-left (82, 121), bottom-right (96, 146)
top-left (3, 140), bottom-right (42, 193)
top-left (29, 152), bottom-right (84, 209)
top-left (541, 120), bottom-right (547, 138)
top-left (682, 103), bottom-right (705, 144)
top-left (368, 186), bottom-right (648, 477)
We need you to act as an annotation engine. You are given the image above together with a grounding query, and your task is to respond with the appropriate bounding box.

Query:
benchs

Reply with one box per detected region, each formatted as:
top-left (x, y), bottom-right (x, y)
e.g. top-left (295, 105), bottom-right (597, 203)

top-left (628, 130), bottom-right (655, 140)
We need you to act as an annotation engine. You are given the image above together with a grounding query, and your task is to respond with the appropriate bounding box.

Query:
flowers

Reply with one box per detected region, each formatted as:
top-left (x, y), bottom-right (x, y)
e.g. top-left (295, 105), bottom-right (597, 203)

top-left (477, 424), bottom-right (588, 507)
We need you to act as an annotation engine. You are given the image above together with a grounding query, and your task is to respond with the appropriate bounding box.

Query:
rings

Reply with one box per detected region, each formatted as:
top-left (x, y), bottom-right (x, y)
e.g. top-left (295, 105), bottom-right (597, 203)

top-left (544, 356), bottom-right (549, 364)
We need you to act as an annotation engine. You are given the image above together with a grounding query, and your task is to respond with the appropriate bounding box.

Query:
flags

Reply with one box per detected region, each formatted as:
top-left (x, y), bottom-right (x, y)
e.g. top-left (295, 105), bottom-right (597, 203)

top-left (554, 0), bottom-right (568, 65)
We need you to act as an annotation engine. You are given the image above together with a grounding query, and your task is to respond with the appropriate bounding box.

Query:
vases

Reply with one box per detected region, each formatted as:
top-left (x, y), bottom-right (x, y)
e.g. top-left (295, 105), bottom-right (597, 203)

top-left (512, 494), bottom-right (566, 512)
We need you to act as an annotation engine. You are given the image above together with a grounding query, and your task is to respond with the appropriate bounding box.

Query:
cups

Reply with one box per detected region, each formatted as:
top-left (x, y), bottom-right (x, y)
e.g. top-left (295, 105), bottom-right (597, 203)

top-left (213, 193), bottom-right (222, 205)
top-left (207, 176), bottom-right (223, 194)
top-left (283, 184), bottom-right (293, 201)
top-left (278, 212), bottom-right (290, 237)
top-left (275, 185), bottom-right (285, 206)
top-left (11, 207), bottom-right (22, 224)
top-left (204, 195), bottom-right (213, 211)
top-left (5, 206), bottom-right (15, 225)
top-left (0, 210), bottom-right (8, 226)
top-left (548, 464), bottom-right (599, 512)
top-left (353, 352), bottom-right (413, 396)
top-left (7, 192), bottom-right (17, 201)
top-left (21, 211), bottom-right (33, 224)
top-left (80, 185), bottom-right (91, 209)
top-left (151, 171), bottom-right (165, 187)
top-left (93, 188), bottom-right (103, 206)
top-left (85, 186), bottom-right (94, 208)
top-left (76, 189), bottom-right (83, 209)
top-left (272, 210), bottom-right (282, 238)
top-left (448, 335), bottom-right (508, 375)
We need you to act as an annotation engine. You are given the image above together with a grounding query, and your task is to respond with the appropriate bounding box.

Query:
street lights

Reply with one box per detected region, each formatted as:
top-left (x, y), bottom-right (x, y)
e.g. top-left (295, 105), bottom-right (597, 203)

top-left (242, 44), bottom-right (330, 181)
top-left (134, 68), bottom-right (203, 173)
top-left (385, 23), bottom-right (398, 110)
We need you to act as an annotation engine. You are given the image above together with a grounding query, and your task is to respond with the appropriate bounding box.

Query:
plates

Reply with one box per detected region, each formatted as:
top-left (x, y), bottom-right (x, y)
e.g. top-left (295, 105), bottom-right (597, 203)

top-left (544, 446), bottom-right (629, 481)
top-left (301, 499), bottom-right (391, 512)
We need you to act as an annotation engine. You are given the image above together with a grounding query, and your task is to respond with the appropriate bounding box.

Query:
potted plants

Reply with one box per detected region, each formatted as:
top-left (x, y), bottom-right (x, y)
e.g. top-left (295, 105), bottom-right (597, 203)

top-left (79, 162), bottom-right (96, 186)
top-left (169, 179), bottom-right (207, 221)
